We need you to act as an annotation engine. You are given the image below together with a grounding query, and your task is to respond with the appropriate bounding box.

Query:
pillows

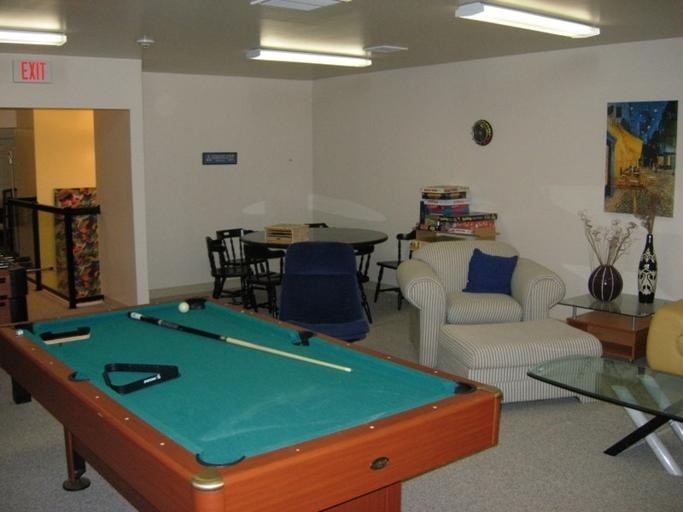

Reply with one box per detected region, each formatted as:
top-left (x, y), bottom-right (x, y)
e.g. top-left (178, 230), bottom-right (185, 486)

top-left (462, 246), bottom-right (518, 294)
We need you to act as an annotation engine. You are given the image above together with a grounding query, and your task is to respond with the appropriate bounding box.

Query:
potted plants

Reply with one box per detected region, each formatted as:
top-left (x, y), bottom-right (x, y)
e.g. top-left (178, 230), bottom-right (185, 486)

top-left (578, 211), bottom-right (637, 302)
top-left (634, 171), bottom-right (659, 303)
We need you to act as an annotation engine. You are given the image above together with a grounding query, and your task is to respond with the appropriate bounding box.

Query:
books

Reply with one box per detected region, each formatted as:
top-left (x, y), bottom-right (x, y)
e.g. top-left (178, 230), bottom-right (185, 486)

top-left (419, 186), bottom-right (498, 235)
top-left (264, 223), bottom-right (310, 231)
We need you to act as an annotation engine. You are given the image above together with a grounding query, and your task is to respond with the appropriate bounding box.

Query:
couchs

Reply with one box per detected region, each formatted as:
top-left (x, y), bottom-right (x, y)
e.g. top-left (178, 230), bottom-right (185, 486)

top-left (398, 240), bottom-right (602, 403)
top-left (279, 242), bottom-right (369, 342)
top-left (647, 299), bottom-right (683, 375)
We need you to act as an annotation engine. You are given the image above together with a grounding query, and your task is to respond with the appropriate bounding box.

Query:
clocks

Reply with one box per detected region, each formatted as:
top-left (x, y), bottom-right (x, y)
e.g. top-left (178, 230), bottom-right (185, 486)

top-left (472, 120), bottom-right (492, 146)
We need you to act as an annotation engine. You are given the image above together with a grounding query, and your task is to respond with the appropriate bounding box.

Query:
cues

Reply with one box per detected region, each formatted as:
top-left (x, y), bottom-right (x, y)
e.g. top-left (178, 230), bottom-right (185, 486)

top-left (127, 311), bottom-right (353, 373)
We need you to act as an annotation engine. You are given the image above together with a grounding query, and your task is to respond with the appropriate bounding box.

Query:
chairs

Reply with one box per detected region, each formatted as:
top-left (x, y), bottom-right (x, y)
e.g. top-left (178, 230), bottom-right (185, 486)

top-left (353, 246), bottom-right (375, 324)
top-left (244, 245), bottom-right (285, 318)
top-left (216, 228), bottom-right (269, 272)
top-left (206, 236), bottom-right (244, 299)
top-left (374, 230), bottom-right (416, 308)
